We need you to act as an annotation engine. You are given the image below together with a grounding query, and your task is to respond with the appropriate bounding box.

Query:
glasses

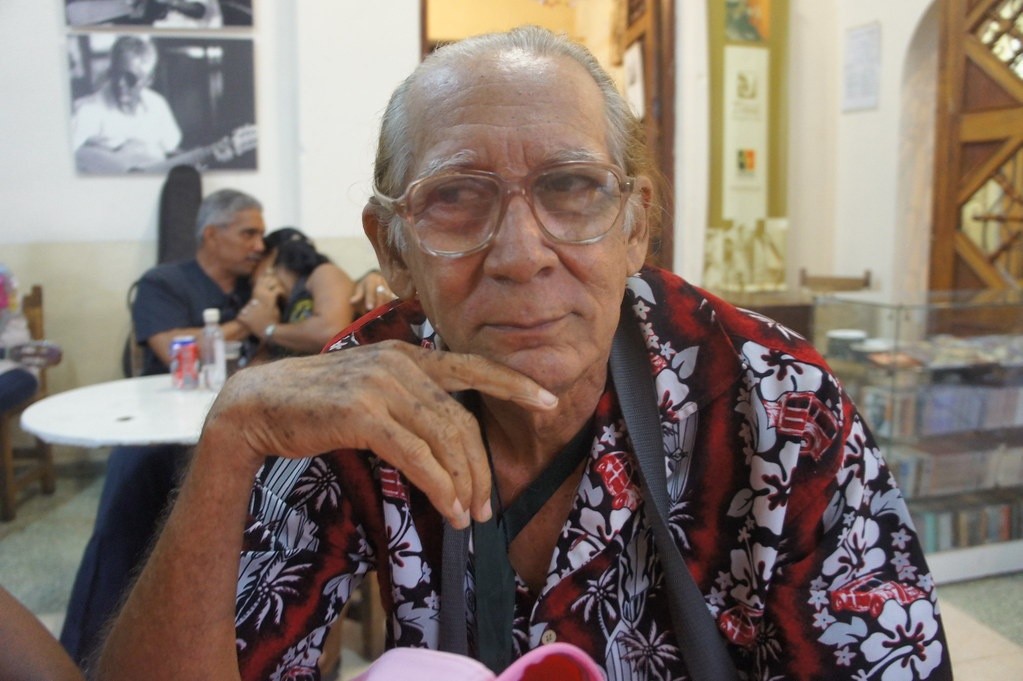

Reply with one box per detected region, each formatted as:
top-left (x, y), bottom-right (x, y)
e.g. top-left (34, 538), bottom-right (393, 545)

top-left (371, 158), bottom-right (639, 260)
top-left (108, 70), bottom-right (147, 88)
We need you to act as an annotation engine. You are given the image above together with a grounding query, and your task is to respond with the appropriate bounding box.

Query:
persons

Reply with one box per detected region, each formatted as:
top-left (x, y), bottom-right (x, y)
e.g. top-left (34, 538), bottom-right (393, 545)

top-left (0, 273), bottom-right (41, 411)
top-left (72, 35), bottom-right (233, 177)
top-left (91, 22), bottom-right (955, 681)
top-left (58, 189), bottom-right (398, 681)
top-left (0, 581), bottom-right (88, 681)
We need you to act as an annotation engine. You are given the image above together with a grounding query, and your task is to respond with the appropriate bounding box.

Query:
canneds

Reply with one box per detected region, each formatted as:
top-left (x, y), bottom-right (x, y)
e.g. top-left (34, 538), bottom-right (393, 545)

top-left (168, 335), bottom-right (200, 391)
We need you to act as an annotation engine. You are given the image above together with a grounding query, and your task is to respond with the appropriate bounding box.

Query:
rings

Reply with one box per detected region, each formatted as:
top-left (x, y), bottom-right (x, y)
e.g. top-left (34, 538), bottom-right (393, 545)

top-left (376, 285), bottom-right (386, 295)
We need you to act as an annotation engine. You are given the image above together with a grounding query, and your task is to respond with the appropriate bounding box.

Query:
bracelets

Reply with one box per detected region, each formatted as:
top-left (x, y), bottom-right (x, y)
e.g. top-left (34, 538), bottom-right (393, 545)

top-left (264, 323), bottom-right (278, 343)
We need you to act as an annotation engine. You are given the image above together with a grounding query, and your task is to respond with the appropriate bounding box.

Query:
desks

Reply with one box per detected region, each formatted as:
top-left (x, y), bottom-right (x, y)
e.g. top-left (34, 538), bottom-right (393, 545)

top-left (19, 373), bottom-right (218, 448)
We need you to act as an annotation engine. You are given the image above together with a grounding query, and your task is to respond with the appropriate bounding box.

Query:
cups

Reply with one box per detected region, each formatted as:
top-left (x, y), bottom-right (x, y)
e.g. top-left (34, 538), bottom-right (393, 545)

top-left (827, 329), bottom-right (867, 363)
top-left (224, 340), bottom-right (243, 379)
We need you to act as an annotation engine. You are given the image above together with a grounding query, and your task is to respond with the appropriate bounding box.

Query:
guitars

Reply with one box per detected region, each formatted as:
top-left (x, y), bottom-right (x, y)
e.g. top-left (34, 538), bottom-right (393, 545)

top-left (74, 122), bottom-right (258, 177)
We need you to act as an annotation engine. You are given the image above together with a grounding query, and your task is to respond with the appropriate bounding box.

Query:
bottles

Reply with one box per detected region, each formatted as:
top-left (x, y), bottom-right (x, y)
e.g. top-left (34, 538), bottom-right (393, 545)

top-left (200, 307), bottom-right (228, 395)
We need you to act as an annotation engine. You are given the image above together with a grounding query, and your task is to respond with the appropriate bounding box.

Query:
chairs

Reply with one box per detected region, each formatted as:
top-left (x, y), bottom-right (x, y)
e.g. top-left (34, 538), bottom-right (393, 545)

top-left (0, 284), bottom-right (57, 525)
